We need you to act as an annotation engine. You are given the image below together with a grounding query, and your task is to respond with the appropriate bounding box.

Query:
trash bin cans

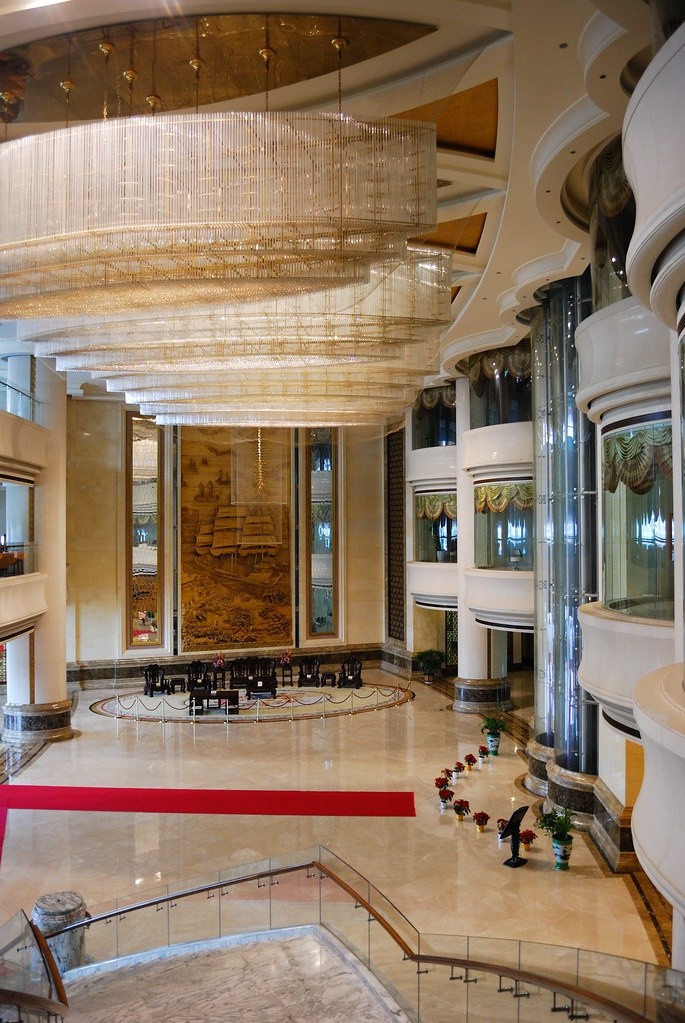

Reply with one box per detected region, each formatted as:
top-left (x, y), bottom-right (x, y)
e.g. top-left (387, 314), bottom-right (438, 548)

top-left (32, 890), bottom-right (87, 977)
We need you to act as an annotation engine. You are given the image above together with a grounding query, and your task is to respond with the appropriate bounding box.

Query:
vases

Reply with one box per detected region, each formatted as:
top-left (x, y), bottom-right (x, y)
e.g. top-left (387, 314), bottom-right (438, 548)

top-left (281, 664), bottom-right (292, 669)
top-left (440, 801), bottom-right (447, 809)
top-left (477, 824), bottom-right (485, 832)
top-left (479, 757), bottom-right (484, 764)
top-left (465, 764), bottom-right (473, 772)
top-left (453, 771), bottom-right (459, 779)
top-left (497, 832), bottom-right (507, 844)
top-left (521, 843), bottom-right (531, 851)
top-left (457, 814), bottom-right (464, 822)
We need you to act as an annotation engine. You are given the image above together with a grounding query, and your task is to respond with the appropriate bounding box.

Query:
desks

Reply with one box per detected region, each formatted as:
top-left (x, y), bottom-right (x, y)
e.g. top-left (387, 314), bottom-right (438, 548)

top-left (282, 667), bottom-right (293, 688)
top-left (205, 669), bottom-right (226, 691)
top-left (188, 689), bottom-right (239, 716)
top-left (171, 678), bottom-right (186, 694)
top-left (321, 673), bottom-right (336, 687)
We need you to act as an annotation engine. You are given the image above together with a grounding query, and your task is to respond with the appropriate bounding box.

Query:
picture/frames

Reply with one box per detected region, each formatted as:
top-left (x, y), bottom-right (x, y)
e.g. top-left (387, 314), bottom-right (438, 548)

top-left (172, 424), bottom-right (301, 659)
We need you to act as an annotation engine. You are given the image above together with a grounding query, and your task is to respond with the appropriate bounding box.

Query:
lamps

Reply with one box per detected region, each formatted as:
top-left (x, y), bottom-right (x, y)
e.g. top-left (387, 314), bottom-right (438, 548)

top-left (0, 16), bottom-right (453, 430)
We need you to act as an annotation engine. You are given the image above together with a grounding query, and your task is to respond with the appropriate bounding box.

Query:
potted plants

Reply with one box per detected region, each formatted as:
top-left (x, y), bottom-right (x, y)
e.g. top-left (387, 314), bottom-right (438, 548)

top-left (413, 648), bottom-right (451, 685)
top-left (479, 716), bottom-right (510, 757)
top-left (533, 800), bottom-right (577, 872)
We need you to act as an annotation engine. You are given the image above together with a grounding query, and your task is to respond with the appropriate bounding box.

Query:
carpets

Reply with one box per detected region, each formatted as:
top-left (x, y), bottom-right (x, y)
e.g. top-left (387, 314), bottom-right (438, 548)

top-left (90, 681), bottom-right (415, 725)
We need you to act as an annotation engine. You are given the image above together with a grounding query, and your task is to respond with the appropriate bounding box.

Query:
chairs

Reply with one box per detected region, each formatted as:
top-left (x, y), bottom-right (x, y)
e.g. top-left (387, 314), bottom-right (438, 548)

top-left (246, 657), bottom-right (279, 700)
top-left (298, 656), bottom-right (320, 688)
top-left (0, 552), bottom-right (27, 579)
top-left (143, 664), bottom-right (172, 697)
top-left (337, 658), bottom-right (363, 690)
top-left (185, 660), bottom-right (210, 691)
top-left (229, 658), bottom-right (247, 690)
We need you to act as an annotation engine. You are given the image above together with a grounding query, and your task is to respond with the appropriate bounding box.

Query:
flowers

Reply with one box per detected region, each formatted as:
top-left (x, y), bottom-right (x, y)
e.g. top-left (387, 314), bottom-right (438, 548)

top-left (454, 761), bottom-right (466, 773)
top-left (519, 829), bottom-right (538, 845)
top-left (439, 789), bottom-right (455, 803)
top-left (452, 799), bottom-right (471, 816)
top-left (497, 819), bottom-right (508, 834)
top-left (473, 811), bottom-right (491, 826)
top-left (434, 777), bottom-right (449, 789)
top-left (464, 753), bottom-right (477, 766)
top-left (277, 653), bottom-right (291, 664)
top-left (478, 745), bottom-right (490, 758)
top-left (441, 768), bottom-right (454, 781)
top-left (211, 658), bottom-right (226, 669)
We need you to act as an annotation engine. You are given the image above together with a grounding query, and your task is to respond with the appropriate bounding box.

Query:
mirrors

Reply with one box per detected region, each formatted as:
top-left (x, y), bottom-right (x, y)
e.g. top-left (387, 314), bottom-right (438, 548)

top-left (303, 428), bottom-right (342, 642)
top-left (124, 409), bottom-right (165, 649)
